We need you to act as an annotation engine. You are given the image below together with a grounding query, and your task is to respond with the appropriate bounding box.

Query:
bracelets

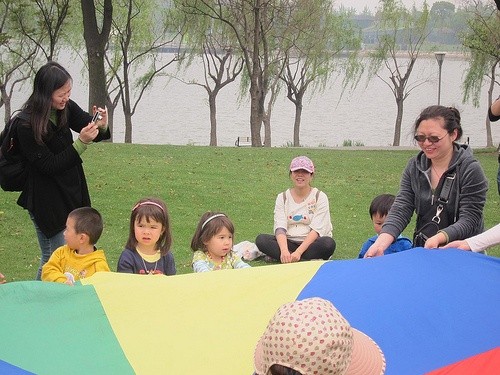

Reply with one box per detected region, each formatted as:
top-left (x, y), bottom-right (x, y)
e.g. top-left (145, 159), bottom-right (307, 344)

top-left (296, 248), bottom-right (302, 255)
top-left (78, 135), bottom-right (94, 145)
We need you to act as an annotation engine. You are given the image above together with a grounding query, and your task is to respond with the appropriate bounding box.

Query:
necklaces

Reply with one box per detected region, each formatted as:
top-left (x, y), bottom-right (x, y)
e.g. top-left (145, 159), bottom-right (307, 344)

top-left (207, 251), bottom-right (225, 271)
top-left (432, 164), bottom-right (440, 178)
top-left (138, 248), bottom-right (158, 275)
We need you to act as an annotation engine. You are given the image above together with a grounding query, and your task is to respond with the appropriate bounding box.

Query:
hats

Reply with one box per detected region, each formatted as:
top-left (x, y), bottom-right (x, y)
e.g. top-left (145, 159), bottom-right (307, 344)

top-left (290, 156), bottom-right (314, 173)
top-left (254, 297), bottom-right (387, 374)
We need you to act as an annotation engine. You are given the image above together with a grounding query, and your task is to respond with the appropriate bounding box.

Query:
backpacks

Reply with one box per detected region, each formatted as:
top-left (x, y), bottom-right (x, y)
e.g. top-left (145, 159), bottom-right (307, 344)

top-left (0, 114), bottom-right (26, 191)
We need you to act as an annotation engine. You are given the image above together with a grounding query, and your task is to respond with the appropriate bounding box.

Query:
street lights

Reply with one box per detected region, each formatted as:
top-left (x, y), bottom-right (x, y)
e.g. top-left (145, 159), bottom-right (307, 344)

top-left (434, 51), bottom-right (446, 107)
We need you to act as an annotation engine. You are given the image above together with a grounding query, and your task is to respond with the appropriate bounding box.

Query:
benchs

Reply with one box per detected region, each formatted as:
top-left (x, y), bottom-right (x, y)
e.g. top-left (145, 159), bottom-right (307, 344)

top-left (235, 137), bottom-right (253, 146)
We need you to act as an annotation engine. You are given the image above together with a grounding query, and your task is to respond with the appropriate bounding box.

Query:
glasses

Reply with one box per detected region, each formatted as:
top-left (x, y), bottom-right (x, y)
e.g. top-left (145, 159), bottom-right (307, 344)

top-left (414, 131), bottom-right (450, 143)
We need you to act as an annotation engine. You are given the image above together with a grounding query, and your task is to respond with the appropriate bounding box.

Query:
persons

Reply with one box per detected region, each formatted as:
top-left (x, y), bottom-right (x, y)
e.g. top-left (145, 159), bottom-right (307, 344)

top-left (41, 207), bottom-right (110, 284)
top-left (117, 197), bottom-right (176, 276)
top-left (363, 105), bottom-right (486, 255)
top-left (255, 156), bottom-right (336, 264)
top-left (357, 194), bottom-right (412, 259)
top-left (488, 95), bottom-right (500, 204)
top-left (252, 298), bottom-right (386, 375)
top-left (439, 223), bottom-right (500, 252)
top-left (16, 62), bottom-right (112, 281)
top-left (191, 212), bottom-right (251, 273)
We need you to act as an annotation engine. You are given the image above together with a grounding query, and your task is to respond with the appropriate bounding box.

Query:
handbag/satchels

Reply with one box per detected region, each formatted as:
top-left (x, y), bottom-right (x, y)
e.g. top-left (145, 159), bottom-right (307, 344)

top-left (413, 170), bottom-right (457, 247)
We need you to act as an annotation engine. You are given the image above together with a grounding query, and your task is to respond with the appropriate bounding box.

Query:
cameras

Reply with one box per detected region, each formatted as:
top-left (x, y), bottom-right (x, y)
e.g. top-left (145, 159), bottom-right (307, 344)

top-left (90, 110), bottom-right (103, 124)
